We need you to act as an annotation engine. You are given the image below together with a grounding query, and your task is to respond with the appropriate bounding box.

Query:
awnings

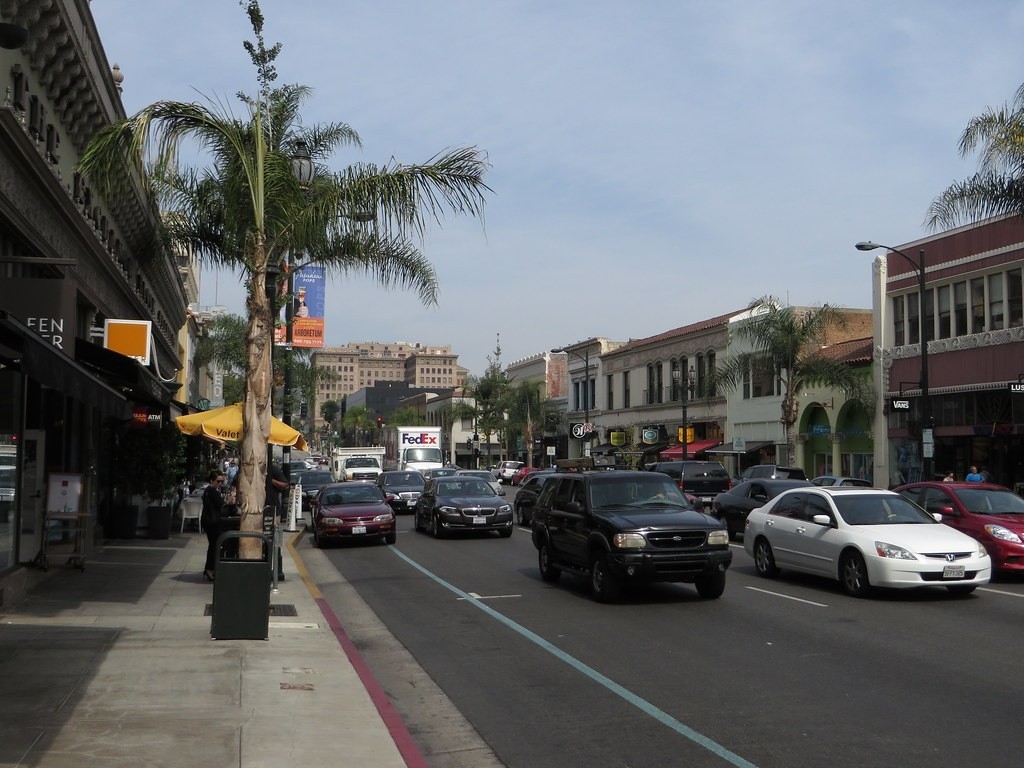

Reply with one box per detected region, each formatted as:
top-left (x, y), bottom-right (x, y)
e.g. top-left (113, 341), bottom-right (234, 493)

top-left (591, 442), bottom-right (630, 454)
top-left (613, 440), bottom-right (668, 457)
top-left (705, 440), bottom-right (774, 454)
top-left (660, 439), bottom-right (721, 459)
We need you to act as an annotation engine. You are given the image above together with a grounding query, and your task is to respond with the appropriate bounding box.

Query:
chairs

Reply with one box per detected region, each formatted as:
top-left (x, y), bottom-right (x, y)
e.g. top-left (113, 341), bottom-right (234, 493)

top-left (482, 475), bottom-right (491, 482)
top-left (440, 484), bottom-right (450, 496)
top-left (327, 494), bottom-right (342, 504)
top-left (462, 484), bottom-right (481, 495)
top-left (159, 481), bottom-right (231, 533)
top-left (408, 476), bottom-right (417, 486)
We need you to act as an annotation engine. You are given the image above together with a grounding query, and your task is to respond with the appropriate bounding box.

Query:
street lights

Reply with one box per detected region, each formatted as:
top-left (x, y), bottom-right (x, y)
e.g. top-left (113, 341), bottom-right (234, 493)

top-left (673, 362), bottom-right (697, 460)
top-left (281, 210), bottom-right (377, 520)
top-left (854, 239), bottom-right (934, 482)
top-left (548, 348), bottom-right (591, 457)
top-left (274, 134), bottom-right (313, 525)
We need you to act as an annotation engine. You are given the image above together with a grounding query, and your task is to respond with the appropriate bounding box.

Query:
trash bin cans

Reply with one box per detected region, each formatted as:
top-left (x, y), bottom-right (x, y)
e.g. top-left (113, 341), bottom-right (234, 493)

top-left (210, 530), bottom-right (273, 640)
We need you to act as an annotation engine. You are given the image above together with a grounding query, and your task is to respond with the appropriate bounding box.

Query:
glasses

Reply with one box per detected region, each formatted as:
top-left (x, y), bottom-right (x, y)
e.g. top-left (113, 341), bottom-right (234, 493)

top-left (215, 480), bottom-right (224, 484)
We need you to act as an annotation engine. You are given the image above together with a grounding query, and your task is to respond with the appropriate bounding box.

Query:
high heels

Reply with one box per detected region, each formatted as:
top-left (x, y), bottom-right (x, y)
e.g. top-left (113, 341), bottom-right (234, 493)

top-left (203, 570), bottom-right (214, 581)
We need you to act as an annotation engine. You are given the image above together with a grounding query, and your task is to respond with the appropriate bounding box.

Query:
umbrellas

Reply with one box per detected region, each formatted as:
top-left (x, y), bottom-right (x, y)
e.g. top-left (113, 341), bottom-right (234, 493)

top-left (173, 401), bottom-right (307, 451)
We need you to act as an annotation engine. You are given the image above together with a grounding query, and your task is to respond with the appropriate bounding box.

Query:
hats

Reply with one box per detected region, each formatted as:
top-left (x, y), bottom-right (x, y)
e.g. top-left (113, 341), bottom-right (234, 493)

top-left (230, 461), bottom-right (235, 464)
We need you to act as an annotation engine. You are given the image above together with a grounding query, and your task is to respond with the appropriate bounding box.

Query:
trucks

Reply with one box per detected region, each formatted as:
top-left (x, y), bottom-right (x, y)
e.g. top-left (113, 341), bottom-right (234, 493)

top-left (0, 443), bottom-right (18, 507)
top-left (326, 445), bottom-right (386, 488)
top-left (395, 425), bottom-right (447, 484)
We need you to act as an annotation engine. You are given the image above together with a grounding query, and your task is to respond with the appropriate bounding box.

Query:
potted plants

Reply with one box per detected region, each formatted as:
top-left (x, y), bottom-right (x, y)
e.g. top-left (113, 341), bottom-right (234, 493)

top-left (102, 415), bottom-right (187, 541)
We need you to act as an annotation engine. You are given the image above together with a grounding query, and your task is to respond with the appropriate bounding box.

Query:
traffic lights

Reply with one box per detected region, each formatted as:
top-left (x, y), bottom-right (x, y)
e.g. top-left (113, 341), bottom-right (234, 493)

top-left (466, 437), bottom-right (472, 451)
top-left (479, 449), bottom-right (483, 460)
top-left (12, 433), bottom-right (17, 440)
top-left (378, 418), bottom-right (383, 428)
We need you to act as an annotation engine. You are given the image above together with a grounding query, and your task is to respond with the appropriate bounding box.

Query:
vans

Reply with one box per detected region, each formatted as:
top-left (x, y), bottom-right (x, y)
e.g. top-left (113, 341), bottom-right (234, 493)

top-left (644, 460), bottom-right (733, 517)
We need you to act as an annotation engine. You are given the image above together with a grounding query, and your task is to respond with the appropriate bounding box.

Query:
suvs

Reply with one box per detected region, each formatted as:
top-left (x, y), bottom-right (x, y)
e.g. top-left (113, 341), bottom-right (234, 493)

top-left (530, 456), bottom-right (733, 599)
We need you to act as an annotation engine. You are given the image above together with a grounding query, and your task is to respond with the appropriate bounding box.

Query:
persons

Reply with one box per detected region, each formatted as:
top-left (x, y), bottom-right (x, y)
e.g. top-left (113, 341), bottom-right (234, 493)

top-left (943, 471), bottom-right (954, 482)
top-left (892, 463), bottom-right (907, 489)
top-left (964, 465), bottom-right (984, 483)
top-left (444, 460), bottom-right (452, 467)
top-left (201, 470), bottom-right (227, 581)
top-left (208, 449), bottom-right (289, 563)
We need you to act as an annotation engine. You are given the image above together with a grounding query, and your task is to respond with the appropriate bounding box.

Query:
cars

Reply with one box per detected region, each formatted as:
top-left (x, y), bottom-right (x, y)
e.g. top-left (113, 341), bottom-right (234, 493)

top-left (743, 485), bottom-right (992, 600)
top-left (451, 467), bottom-right (506, 501)
top-left (291, 453), bottom-right (335, 510)
top-left (309, 481), bottom-right (397, 546)
top-left (886, 481), bottom-right (1024, 578)
top-left (414, 476), bottom-right (514, 538)
top-left (373, 469), bottom-right (428, 512)
top-left (423, 466), bottom-right (460, 488)
top-left (711, 465), bottom-right (874, 540)
top-left (491, 460), bottom-right (566, 528)
